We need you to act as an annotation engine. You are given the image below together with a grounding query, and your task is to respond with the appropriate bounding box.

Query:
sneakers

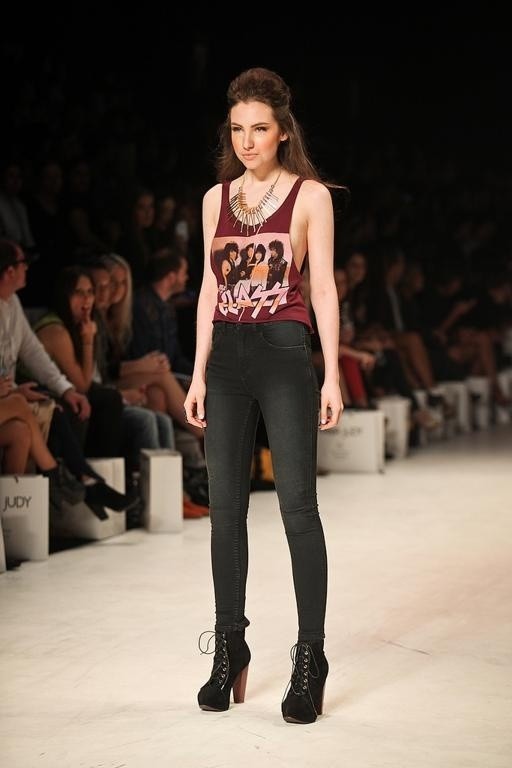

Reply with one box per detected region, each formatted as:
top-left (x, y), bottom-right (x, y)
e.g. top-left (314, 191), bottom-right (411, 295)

top-left (412, 408), bottom-right (440, 430)
top-left (182, 477), bottom-right (276, 519)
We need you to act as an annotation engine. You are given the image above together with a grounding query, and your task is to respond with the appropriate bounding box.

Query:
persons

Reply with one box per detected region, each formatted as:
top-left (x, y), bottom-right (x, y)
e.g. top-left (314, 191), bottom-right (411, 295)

top-left (180, 68), bottom-right (343, 723)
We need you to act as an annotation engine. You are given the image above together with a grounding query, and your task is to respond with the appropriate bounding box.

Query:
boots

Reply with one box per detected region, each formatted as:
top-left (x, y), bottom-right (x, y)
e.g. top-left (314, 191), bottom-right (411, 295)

top-left (37, 463), bottom-right (85, 509)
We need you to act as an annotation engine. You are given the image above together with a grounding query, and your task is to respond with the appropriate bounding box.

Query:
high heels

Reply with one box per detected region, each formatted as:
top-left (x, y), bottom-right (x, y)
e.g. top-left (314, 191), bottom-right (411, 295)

top-left (81, 479), bottom-right (140, 520)
top-left (197, 626), bottom-right (251, 711)
top-left (280, 639), bottom-right (329, 724)
